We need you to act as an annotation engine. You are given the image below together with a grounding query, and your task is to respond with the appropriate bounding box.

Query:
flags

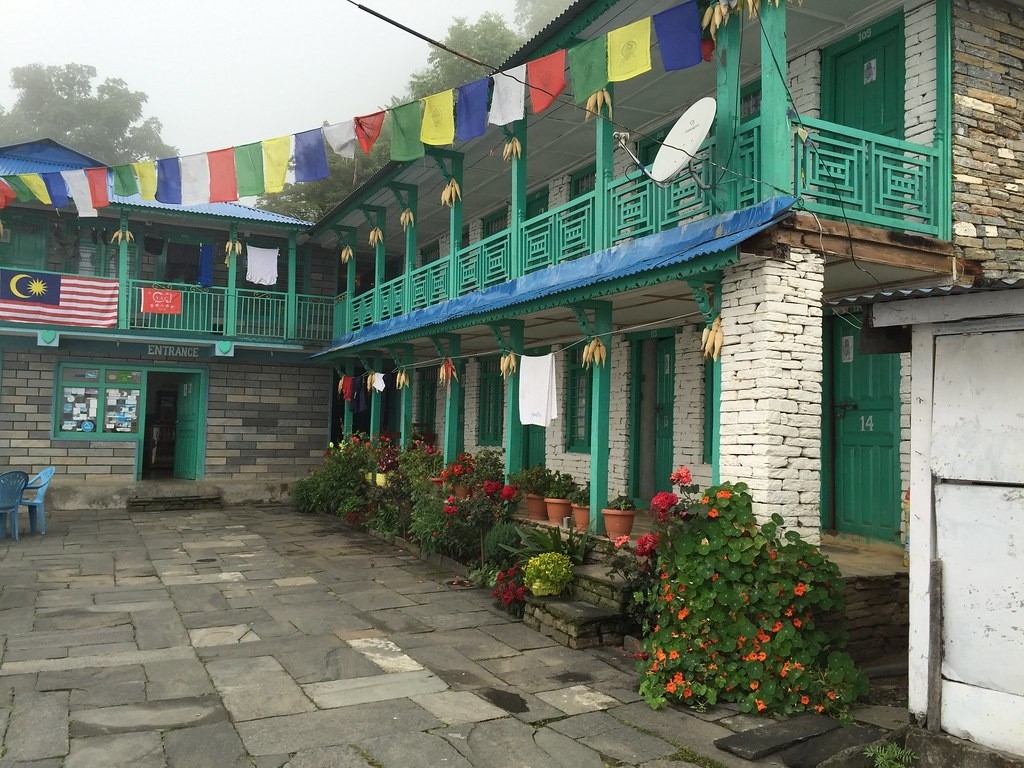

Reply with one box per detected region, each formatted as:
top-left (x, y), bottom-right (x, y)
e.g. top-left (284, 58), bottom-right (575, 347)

top-left (390, 0), bottom-right (703, 163)
top-left (0, 110), bottom-right (390, 218)
top-left (0, 268), bottom-right (120, 329)
top-left (140, 287), bottom-right (182, 314)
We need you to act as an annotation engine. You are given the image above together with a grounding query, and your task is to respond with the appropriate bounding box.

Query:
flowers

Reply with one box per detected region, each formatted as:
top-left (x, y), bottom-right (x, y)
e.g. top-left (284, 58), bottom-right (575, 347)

top-left (288, 422), bottom-right (519, 553)
top-left (490, 562), bottom-right (529, 614)
top-left (605, 465), bottom-right (873, 719)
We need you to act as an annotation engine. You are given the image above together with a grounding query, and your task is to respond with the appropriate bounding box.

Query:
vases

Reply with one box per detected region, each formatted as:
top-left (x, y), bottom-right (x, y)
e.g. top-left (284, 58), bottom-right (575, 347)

top-left (455, 482), bottom-right (469, 498)
top-left (430, 476), bottom-right (443, 488)
top-left (364, 471), bottom-right (373, 483)
top-left (375, 472), bottom-right (387, 487)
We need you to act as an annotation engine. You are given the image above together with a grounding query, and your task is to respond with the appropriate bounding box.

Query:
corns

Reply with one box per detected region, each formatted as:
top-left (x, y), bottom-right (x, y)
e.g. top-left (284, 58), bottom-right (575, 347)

top-left (581, 338), bottom-right (606, 368)
top-left (396, 369), bottom-right (410, 389)
top-left (367, 374), bottom-right (375, 391)
top-left (502, 142), bottom-right (521, 160)
top-left (584, 90), bottom-right (613, 120)
top-left (400, 212), bottom-right (414, 228)
top-left (700, 317), bottom-right (723, 362)
top-left (441, 181), bottom-right (461, 206)
top-left (341, 246), bottom-right (354, 264)
top-left (500, 353), bottom-right (517, 380)
top-left (225, 241), bottom-right (242, 254)
top-left (701, 0), bottom-right (780, 36)
top-left (111, 231), bottom-right (135, 245)
top-left (369, 229), bottom-right (384, 245)
top-left (439, 364), bottom-right (458, 385)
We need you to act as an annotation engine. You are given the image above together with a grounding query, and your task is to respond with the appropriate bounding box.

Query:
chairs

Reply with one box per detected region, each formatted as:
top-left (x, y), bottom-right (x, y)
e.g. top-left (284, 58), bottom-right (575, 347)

top-left (10, 466), bottom-right (57, 537)
top-left (0, 469), bottom-right (29, 542)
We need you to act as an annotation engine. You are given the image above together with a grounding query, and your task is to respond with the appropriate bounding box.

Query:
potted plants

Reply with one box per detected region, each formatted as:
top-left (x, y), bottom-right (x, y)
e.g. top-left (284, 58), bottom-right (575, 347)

top-left (507, 464), bottom-right (554, 519)
top-left (543, 472), bottom-right (580, 526)
top-left (524, 552), bottom-right (576, 598)
top-left (569, 480), bottom-right (591, 530)
top-left (601, 497), bottom-right (638, 540)
